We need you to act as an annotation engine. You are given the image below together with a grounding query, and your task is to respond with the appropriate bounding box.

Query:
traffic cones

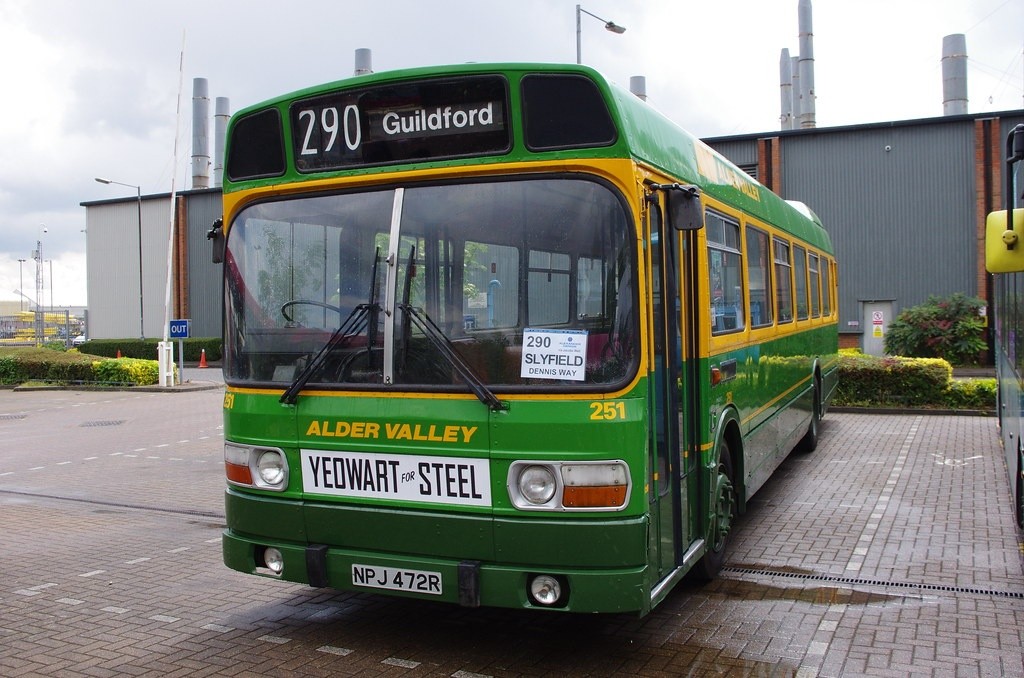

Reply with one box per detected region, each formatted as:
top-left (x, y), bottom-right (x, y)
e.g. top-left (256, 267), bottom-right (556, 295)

top-left (198, 349), bottom-right (209, 368)
top-left (116, 349), bottom-right (122, 359)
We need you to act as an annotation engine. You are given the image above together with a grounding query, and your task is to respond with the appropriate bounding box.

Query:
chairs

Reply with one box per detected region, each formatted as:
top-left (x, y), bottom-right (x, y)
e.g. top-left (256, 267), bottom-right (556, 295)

top-left (583, 331), bottom-right (621, 372)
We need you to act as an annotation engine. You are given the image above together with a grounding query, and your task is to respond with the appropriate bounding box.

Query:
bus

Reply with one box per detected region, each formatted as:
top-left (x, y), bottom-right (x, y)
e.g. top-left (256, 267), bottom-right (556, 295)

top-left (200, 60), bottom-right (841, 624)
top-left (984, 125), bottom-right (1024, 525)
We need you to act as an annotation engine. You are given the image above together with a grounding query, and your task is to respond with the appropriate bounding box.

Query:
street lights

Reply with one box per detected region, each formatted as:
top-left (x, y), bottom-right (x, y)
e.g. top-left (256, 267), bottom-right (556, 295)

top-left (574, 3), bottom-right (627, 66)
top-left (17, 257), bottom-right (27, 312)
top-left (44, 259), bottom-right (54, 312)
top-left (94, 177), bottom-right (146, 342)
